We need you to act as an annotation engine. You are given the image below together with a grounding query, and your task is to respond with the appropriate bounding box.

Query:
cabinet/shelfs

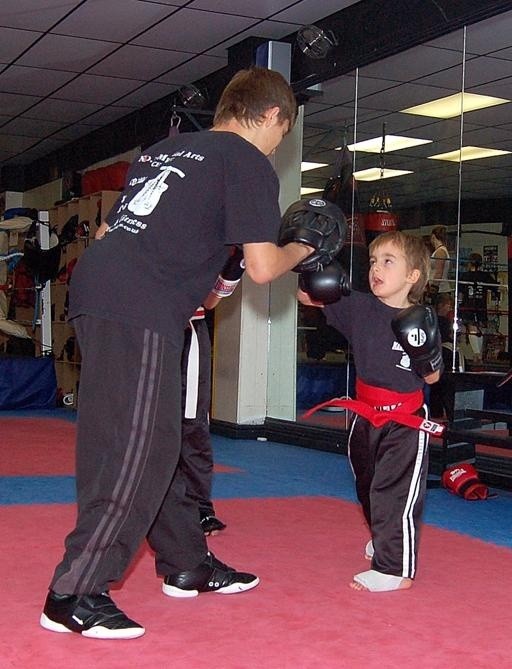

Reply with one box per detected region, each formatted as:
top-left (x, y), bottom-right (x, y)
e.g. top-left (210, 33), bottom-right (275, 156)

top-left (50, 191), bottom-right (122, 412)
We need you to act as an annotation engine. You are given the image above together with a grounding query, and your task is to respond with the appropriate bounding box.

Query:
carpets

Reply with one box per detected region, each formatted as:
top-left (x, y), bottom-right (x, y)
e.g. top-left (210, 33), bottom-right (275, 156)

top-left (0, 495), bottom-right (512, 669)
top-left (0, 417), bottom-right (245, 476)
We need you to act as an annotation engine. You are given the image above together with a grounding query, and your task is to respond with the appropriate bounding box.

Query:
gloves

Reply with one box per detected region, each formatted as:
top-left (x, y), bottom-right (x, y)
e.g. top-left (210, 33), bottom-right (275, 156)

top-left (211, 243), bottom-right (246, 297)
top-left (277, 198), bottom-right (352, 305)
top-left (441, 463), bottom-right (489, 501)
top-left (391, 305), bottom-right (443, 378)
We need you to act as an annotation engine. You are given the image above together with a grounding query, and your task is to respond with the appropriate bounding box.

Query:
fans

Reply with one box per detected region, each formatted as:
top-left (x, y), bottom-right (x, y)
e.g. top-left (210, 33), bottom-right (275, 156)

top-left (297, 25), bottom-right (338, 60)
top-left (177, 83), bottom-right (209, 108)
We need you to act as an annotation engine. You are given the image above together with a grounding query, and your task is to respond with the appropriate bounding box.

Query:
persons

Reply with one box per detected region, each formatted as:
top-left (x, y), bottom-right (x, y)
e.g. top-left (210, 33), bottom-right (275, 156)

top-left (427, 225), bottom-right (451, 317)
top-left (40, 66), bottom-right (317, 641)
top-left (458, 253), bottom-right (500, 366)
top-left (298, 231), bottom-right (444, 592)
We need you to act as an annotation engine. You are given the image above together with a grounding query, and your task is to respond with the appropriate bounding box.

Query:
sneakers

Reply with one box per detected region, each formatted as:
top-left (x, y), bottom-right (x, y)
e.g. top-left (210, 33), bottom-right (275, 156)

top-left (198, 501), bottom-right (227, 535)
top-left (40, 586), bottom-right (145, 640)
top-left (162, 551), bottom-right (259, 598)
